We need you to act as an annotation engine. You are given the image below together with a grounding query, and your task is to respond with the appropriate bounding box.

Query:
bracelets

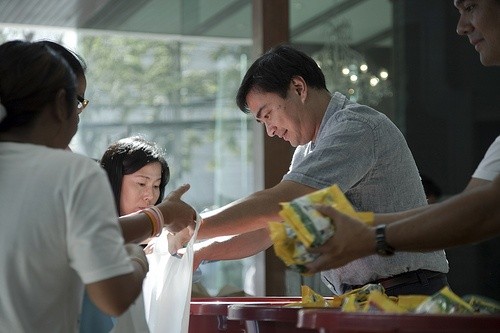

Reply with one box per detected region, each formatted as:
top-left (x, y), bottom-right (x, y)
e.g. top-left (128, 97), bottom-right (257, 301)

top-left (141, 206), bottom-right (164, 237)
top-left (126, 256), bottom-right (147, 277)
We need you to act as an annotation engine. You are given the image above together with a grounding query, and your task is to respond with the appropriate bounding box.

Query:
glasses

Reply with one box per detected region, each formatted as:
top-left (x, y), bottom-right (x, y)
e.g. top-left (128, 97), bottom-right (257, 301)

top-left (76, 93), bottom-right (89, 114)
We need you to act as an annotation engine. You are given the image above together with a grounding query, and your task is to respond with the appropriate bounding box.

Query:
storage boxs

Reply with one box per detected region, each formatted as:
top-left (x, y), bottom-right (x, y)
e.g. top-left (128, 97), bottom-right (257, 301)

top-left (190, 297), bottom-right (500, 333)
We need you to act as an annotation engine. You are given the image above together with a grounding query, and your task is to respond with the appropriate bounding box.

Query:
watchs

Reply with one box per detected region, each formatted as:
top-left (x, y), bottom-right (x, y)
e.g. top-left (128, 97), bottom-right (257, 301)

top-left (376, 223), bottom-right (394, 256)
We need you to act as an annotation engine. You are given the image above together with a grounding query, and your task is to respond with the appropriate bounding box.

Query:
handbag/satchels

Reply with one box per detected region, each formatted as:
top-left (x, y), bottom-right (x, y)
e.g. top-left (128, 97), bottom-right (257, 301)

top-left (142, 208), bottom-right (202, 333)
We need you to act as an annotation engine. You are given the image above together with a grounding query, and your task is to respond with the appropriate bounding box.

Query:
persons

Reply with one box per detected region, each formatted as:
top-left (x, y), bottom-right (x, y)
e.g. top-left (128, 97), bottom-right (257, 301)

top-left (81, 136), bottom-right (170, 333)
top-left (41, 41), bottom-right (203, 245)
top-left (304, 0), bottom-right (500, 275)
top-left (0, 39), bottom-right (149, 333)
top-left (144, 46), bottom-right (450, 295)
